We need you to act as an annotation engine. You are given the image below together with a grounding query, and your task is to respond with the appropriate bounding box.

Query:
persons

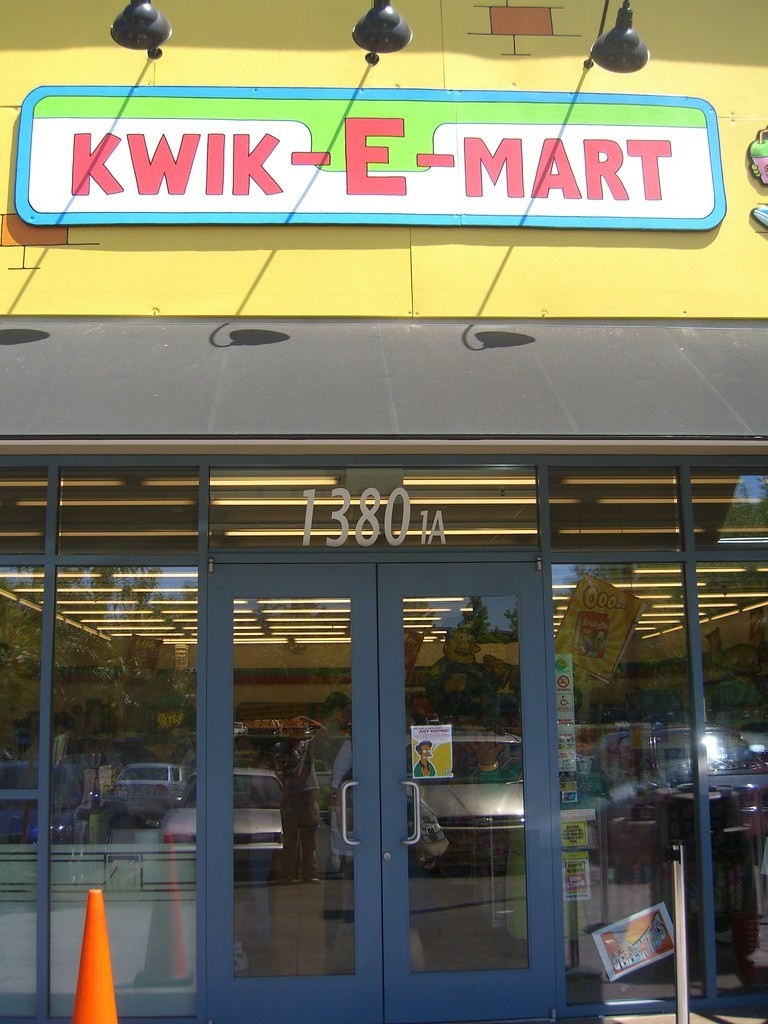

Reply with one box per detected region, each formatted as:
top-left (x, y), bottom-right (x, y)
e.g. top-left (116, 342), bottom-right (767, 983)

top-left (15, 709), bottom-right (125, 824)
top-left (274, 716), bottom-right (329, 884)
top-left (309, 693), bottom-right (354, 949)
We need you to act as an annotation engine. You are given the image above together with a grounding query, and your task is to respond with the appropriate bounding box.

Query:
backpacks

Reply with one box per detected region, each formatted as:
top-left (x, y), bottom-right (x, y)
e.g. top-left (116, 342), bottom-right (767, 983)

top-left (407, 786), bottom-right (449, 867)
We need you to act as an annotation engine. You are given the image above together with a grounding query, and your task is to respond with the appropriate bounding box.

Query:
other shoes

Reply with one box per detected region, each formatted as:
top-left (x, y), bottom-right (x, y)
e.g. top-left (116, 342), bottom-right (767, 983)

top-left (278, 876), bottom-right (293, 884)
top-left (303, 877), bottom-right (320, 883)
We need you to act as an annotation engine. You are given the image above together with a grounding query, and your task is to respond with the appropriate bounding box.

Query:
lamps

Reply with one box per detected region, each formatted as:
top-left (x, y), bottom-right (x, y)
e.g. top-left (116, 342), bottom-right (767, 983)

top-left (583, 0), bottom-right (650, 73)
top-left (111, 0), bottom-right (172, 59)
top-left (352, 0), bottom-right (414, 64)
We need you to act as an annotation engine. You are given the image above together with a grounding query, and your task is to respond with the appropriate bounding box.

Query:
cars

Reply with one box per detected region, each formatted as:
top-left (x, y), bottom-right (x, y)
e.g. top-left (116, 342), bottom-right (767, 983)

top-left (105, 762), bottom-right (192, 824)
top-left (0, 759), bottom-right (83, 843)
top-left (586, 723), bottom-right (768, 838)
top-left (158, 769), bottom-right (294, 885)
top-left (233, 721), bottom-right (249, 735)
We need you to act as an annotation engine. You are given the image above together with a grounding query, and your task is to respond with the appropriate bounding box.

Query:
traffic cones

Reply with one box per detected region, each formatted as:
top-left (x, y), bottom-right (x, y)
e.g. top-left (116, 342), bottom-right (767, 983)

top-left (70, 889), bottom-right (118, 1024)
top-left (128, 832), bottom-right (195, 987)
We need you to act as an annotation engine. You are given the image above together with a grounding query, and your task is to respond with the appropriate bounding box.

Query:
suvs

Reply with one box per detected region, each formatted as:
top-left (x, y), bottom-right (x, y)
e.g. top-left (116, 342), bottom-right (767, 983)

top-left (404, 726), bottom-right (527, 877)
